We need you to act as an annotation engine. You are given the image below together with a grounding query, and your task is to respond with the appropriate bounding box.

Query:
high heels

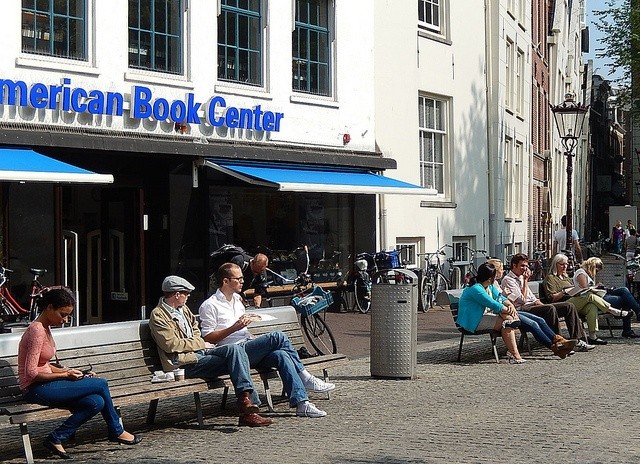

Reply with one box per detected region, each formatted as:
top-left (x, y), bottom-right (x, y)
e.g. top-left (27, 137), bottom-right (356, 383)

top-left (507, 350), bottom-right (527, 364)
top-left (502, 319), bottom-right (522, 330)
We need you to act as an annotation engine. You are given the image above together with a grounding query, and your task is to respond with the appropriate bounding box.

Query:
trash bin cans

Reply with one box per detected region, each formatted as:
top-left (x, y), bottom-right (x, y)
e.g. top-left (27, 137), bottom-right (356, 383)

top-left (595, 253), bottom-right (628, 330)
top-left (371, 268), bottom-right (418, 380)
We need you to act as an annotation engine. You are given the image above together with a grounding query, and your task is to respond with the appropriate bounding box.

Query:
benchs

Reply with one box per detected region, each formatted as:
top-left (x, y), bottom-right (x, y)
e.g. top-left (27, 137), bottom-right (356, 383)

top-left (437, 280), bottom-right (615, 363)
top-left (0, 306), bottom-right (349, 464)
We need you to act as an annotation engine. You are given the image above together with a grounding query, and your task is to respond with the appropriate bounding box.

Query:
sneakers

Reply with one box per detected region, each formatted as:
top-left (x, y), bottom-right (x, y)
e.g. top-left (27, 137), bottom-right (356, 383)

top-left (303, 374), bottom-right (335, 393)
top-left (583, 341), bottom-right (596, 351)
top-left (296, 403), bottom-right (327, 418)
top-left (572, 341), bottom-right (590, 352)
top-left (622, 330), bottom-right (638, 338)
top-left (588, 337), bottom-right (607, 345)
top-left (637, 312), bottom-right (640, 321)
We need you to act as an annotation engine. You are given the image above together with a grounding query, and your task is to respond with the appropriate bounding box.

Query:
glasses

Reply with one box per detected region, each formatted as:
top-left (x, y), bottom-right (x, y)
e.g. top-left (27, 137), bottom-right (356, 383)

top-left (229, 277), bottom-right (244, 283)
top-left (557, 262), bottom-right (569, 268)
top-left (253, 271), bottom-right (262, 276)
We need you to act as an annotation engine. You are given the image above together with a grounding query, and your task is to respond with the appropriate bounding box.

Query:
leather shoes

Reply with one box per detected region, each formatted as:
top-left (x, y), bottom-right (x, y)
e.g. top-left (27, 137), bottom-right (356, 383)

top-left (110, 429), bottom-right (143, 445)
top-left (239, 412), bottom-right (272, 427)
top-left (613, 310), bottom-right (633, 319)
top-left (233, 400), bottom-right (259, 417)
top-left (43, 438), bottom-right (70, 459)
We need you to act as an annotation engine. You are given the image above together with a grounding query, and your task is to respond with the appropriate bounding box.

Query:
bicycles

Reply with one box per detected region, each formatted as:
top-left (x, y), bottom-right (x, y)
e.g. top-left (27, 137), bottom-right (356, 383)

top-left (352, 252), bottom-right (384, 314)
top-left (461, 244), bottom-right (507, 290)
top-left (416, 251), bottom-right (450, 313)
top-left (527, 251), bottom-right (544, 281)
top-left (436, 255), bottom-right (459, 291)
top-left (624, 260), bottom-right (635, 295)
top-left (382, 244), bottom-right (413, 270)
top-left (0, 259), bottom-right (76, 333)
top-left (291, 273), bottom-right (337, 356)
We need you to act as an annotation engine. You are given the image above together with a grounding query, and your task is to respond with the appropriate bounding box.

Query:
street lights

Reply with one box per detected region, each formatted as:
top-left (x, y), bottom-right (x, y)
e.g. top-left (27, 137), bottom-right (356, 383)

top-left (551, 100), bottom-right (591, 279)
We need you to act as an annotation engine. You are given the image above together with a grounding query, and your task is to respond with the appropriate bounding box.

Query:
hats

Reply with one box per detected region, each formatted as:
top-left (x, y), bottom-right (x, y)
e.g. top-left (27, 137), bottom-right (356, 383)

top-left (162, 275), bottom-right (196, 293)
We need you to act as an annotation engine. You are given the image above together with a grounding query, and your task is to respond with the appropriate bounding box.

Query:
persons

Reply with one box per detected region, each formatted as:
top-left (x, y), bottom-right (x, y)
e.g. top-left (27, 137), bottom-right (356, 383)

top-left (625, 219), bottom-right (636, 238)
top-left (197, 261), bottom-right (337, 416)
top-left (149, 274), bottom-right (272, 427)
top-left (613, 220), bottom-right (624, 259)
top-left (537, 253), bottom-right (634, 344)
top-left (208, 252), bottom-right (272, 307)
top-left (459, 262), bottom-right (527, 363)
top-left (16, 290), bottom-right (142, 458)
top-left (574, 257), bottom-right (639, 338)
top-left (482, 258), bottom-right (579, 359)
top-left (624, 228), bottom-right (638, 257)
top-left (552, 213), bottom-right (583, 264)
top-left (498, 252), bottom-right (594, 355)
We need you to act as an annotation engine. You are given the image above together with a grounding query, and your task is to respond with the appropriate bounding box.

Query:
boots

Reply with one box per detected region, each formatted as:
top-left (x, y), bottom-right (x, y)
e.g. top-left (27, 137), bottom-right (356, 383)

top-left (551, 343), bottom-right (575, 359)
top-left (554, 335), bottom-right (579, 347)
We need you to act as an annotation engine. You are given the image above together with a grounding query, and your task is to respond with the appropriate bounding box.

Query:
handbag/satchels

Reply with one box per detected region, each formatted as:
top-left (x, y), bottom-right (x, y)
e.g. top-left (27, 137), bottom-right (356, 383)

top-left (50, 354), bottom-right (65, 369)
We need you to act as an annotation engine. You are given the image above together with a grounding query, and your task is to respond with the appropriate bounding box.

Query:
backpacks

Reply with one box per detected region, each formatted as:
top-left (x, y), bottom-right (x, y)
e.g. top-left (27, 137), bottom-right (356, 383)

top-left (207, 243), bottom-right (250, 276)
